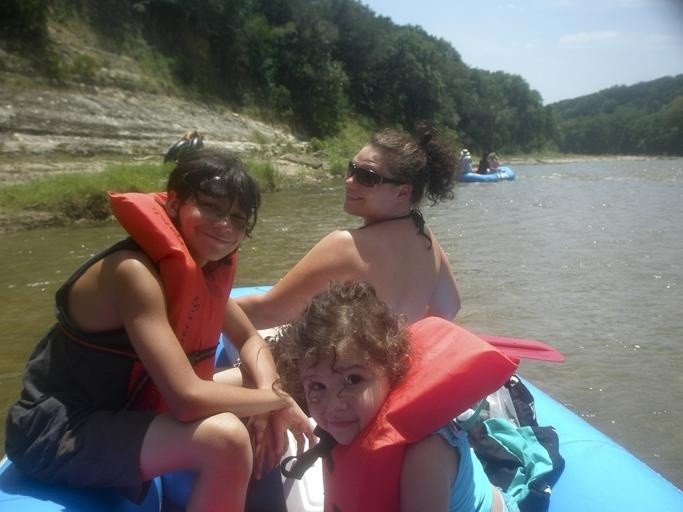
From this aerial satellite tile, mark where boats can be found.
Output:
[456,166,515,182]
[2,283,683,511]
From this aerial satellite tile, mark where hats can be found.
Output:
[461,149,470,156]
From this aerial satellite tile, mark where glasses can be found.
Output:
[199,176,250,210]
[348,160,409,187]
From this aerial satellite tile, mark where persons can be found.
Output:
[459,149,474,173]
[488,152,500,172]
[5,130,320,512]
[476,150,491,174]
[229,122,461,412]
[263,278,525,512]
